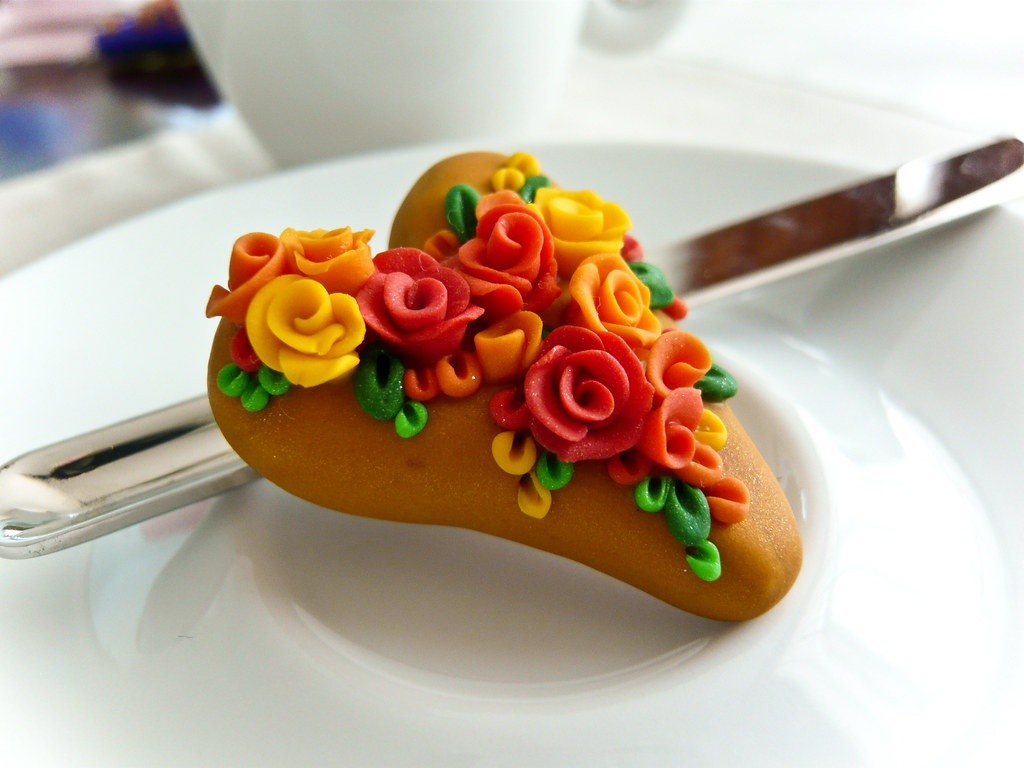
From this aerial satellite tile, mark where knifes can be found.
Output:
[0,138,1024,558]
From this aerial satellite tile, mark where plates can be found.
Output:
[0,149,1024,768]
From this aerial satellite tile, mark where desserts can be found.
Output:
[205,150,802,622]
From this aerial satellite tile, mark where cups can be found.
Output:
[169,1,680,170]
